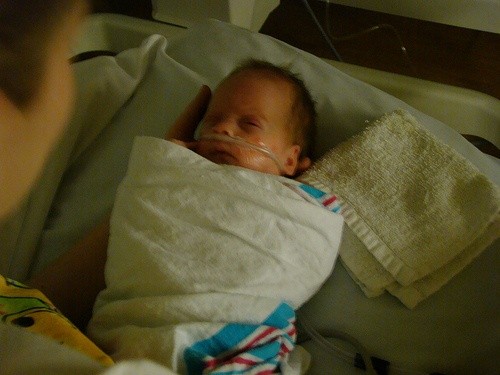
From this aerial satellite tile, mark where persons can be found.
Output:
[0,0,210,373]
[106,54,318,375]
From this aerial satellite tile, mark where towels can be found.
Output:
[296,109,500,309]
